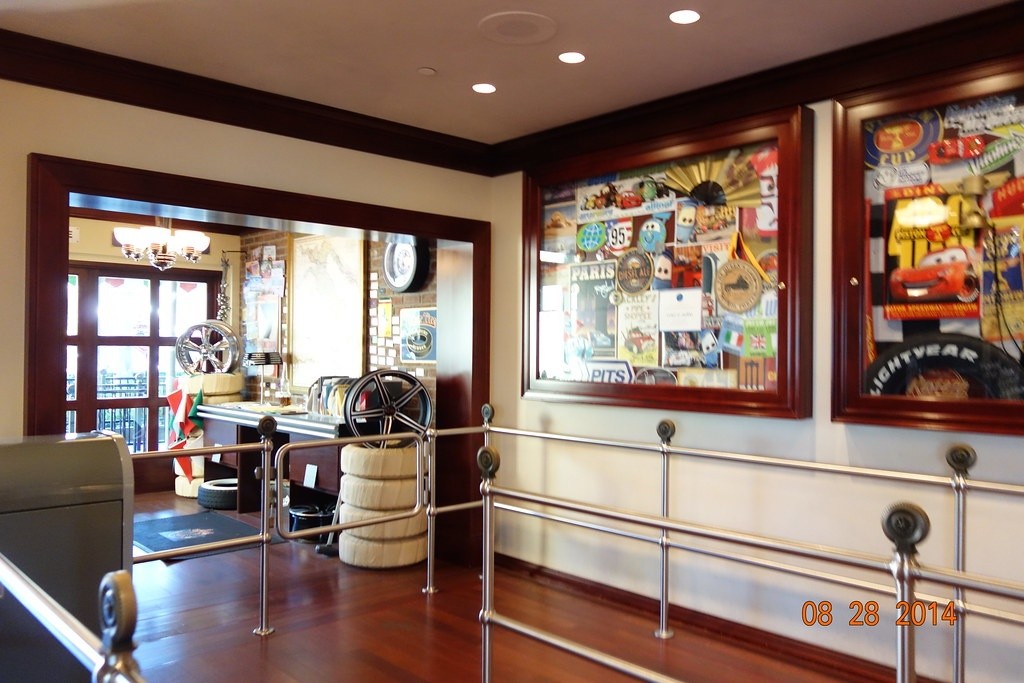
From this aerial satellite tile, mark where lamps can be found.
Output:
[114,225,210,271]
[243,351,282,407]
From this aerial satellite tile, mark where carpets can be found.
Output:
[133,509,289,562]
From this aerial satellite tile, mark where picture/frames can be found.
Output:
[286,225,367,394]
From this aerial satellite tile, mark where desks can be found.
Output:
[196,401,367,532]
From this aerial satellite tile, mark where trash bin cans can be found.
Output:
[0,429,136,683]
